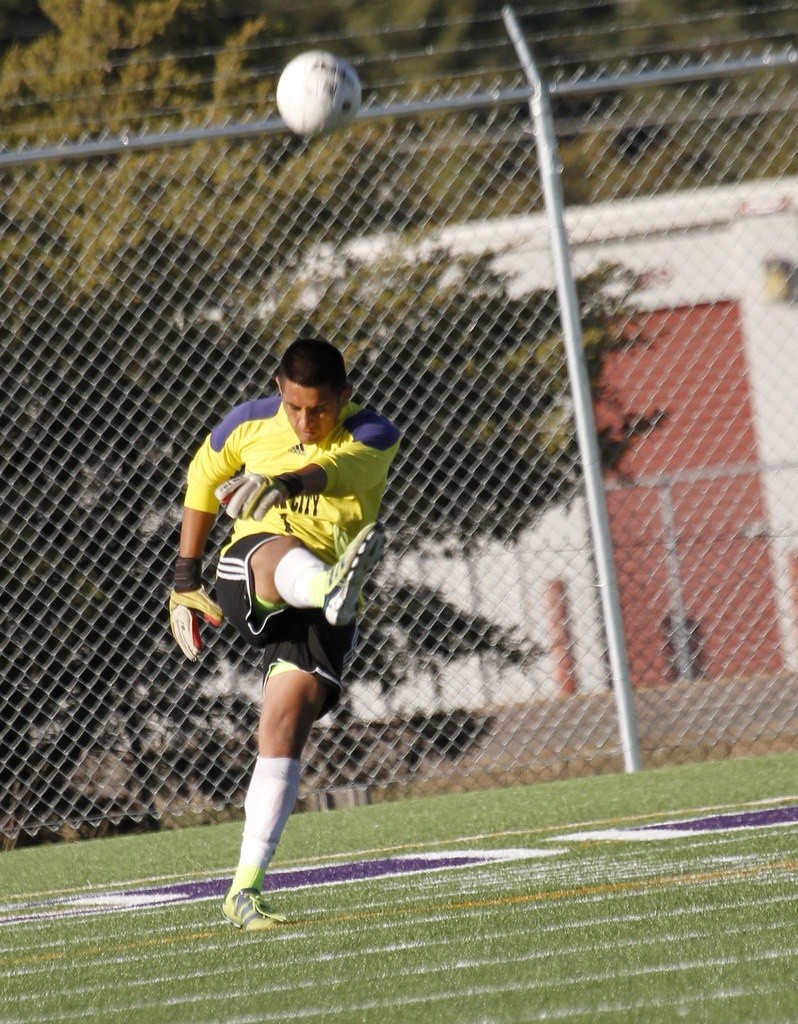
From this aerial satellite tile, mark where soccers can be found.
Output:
[276,49,362,138]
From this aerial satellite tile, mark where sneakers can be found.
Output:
[220,884,290,931]
[323,517,387,625]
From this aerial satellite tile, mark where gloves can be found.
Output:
[215,468,304,521]
[167,559,223,662]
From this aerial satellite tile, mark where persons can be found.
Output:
[169,339,402,931]
